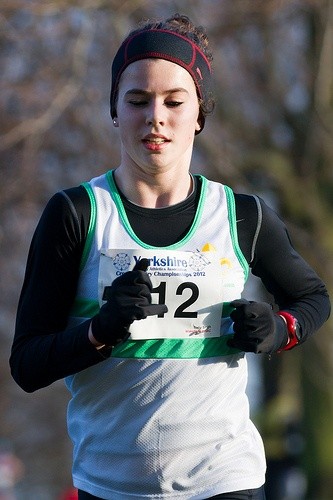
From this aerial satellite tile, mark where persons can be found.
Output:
[8,14,333,500]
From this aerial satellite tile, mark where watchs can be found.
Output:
[278,310,302,350]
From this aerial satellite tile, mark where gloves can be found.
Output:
[224,294,290,357]
[86,254,169,344]
